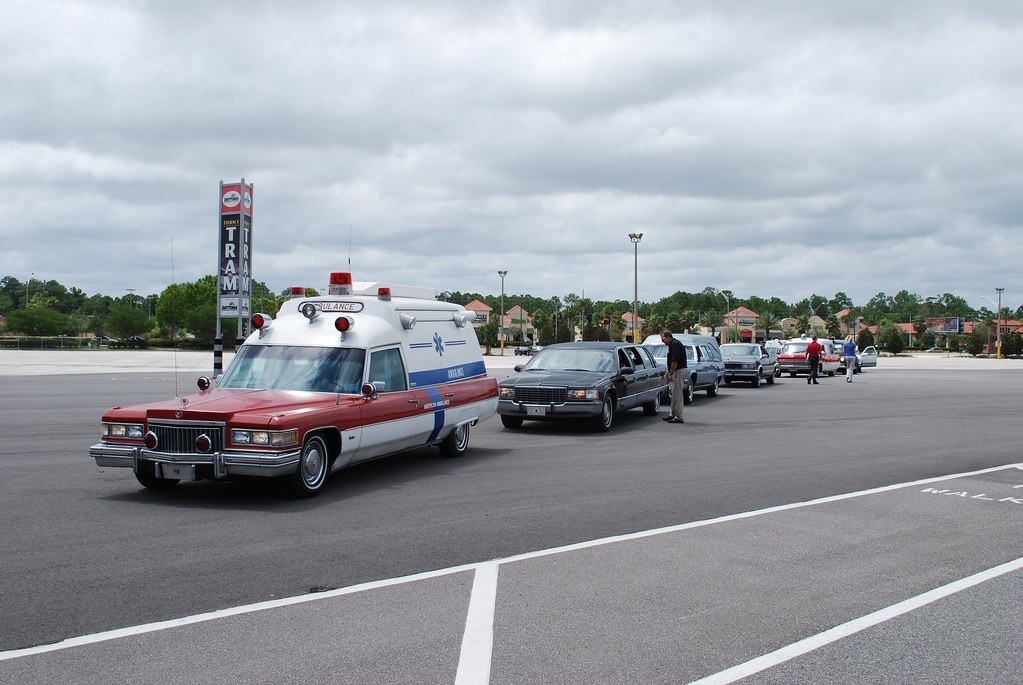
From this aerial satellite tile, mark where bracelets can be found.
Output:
[670,372,673,375]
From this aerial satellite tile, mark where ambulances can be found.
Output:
[88,273,499,501]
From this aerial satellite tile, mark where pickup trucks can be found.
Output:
[641,334,725,407]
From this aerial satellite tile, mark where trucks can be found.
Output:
[765,339,787,357]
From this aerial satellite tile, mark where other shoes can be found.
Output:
[663,416,674,421]
[807,377,811,384]
[846,377,852,382]
[813,381,819,384]
[668,417,684,423]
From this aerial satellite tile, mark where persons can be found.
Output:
[660,331,687,424]
[805,336,823,384]
[843,335,859,383]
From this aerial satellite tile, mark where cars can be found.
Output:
[925,347,943,353]
[89,335,118,346]
[719,343,777,388]
[833,340,877,375]
[777,334,840,377]
[122,336,146,348]
[526,346,543,357]
[497,342,667,433]
[514,346,527,356]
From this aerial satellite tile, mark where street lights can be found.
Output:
[629,233,643,344]
[995,287,1005,359]
[126,289,135,310]
[497,270,508,355]
